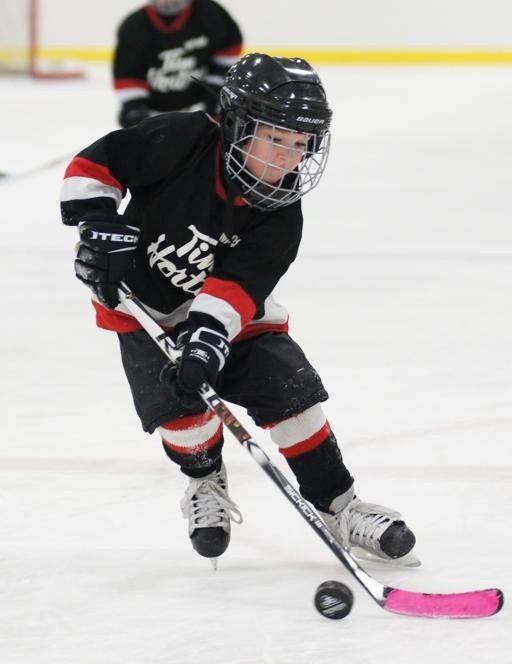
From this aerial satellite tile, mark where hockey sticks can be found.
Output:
[115,282,503,620]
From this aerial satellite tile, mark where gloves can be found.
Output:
[73,217,147,311]
[119,98,149,129]
[157,311,230,409]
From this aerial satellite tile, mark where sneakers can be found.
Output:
[297,475,416,561]
[179,462,243,559]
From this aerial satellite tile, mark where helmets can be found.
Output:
[218,52,334,212]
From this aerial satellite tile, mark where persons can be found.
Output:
[113,0,245,128]
[61,51,418,563]
